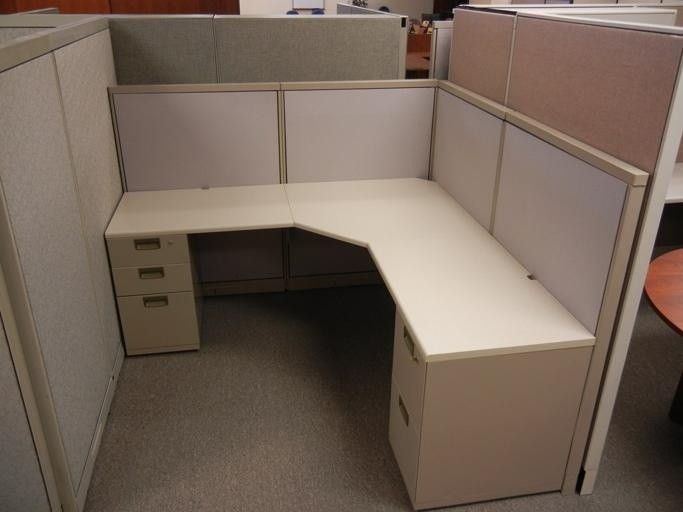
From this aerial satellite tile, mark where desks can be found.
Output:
[281,177,597,509]
[104,182,295,355]
